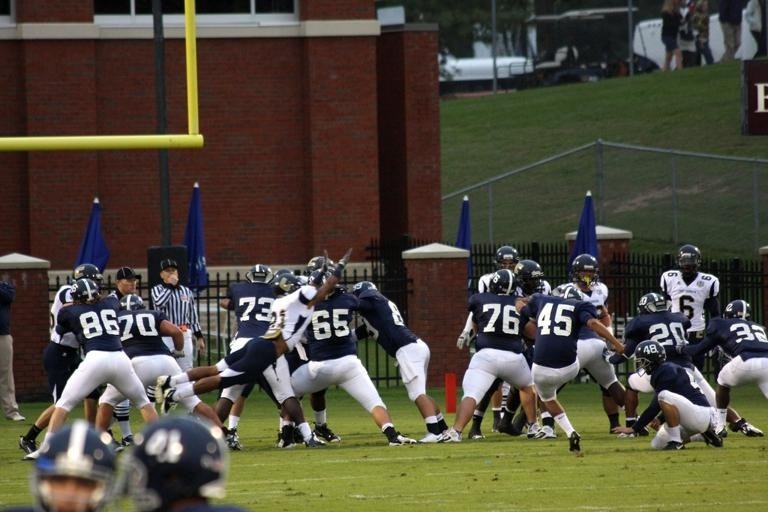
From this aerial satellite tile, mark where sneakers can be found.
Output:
[310,423,342,444]
[18,434,36,453]
[23,442,49,461]
[226,429,244,451]
[154,374,173,403]
[122,433,137,446]
[441,417,651,451]
[106,427,124,453]
[6,412,26,422]
[659,441,687,452]
[160,388,177,416]
[277,423,328,448]
[388,430,416,446]
[417,432,444,443]
[701,418,765,447]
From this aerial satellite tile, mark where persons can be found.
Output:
[437,246,768,452]
[1,273,27,421]
[18,248,453,461]
[10,418,119,511]
[120,413,252,510]
[661,0,767,73]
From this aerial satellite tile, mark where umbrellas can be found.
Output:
[453,196,473,300]
[566,189,598,274]
[181,181,208,294]
[75,198,109,275]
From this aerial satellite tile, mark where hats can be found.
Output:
[114,265,143,281]
[158,258,179,273]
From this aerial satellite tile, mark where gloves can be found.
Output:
[674,339,690,355]
[337,246,354,269]
[321,246,329,274]
[171,347,185,359]
[601,347,621,364]
[456,331,473,350]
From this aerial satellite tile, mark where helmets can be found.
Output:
[70,278,101,301]
[489,268,518,297]
[557,285,584,302]
[636,291,673,315]
[33,422,121,512]
[126,412,230,504]
[307,269,337,296]
[571,252,602,284]
[73,262,104,280]
[351,280,378,295]
[119,292,146,313]
[676,244,703,269]
[244,264,275,285]
[271,268,305,298]
[494,245,519,263]
[722,299,753,321]
[306,255,337,278]
[633,338,668,376]
[513,258,545,297]
